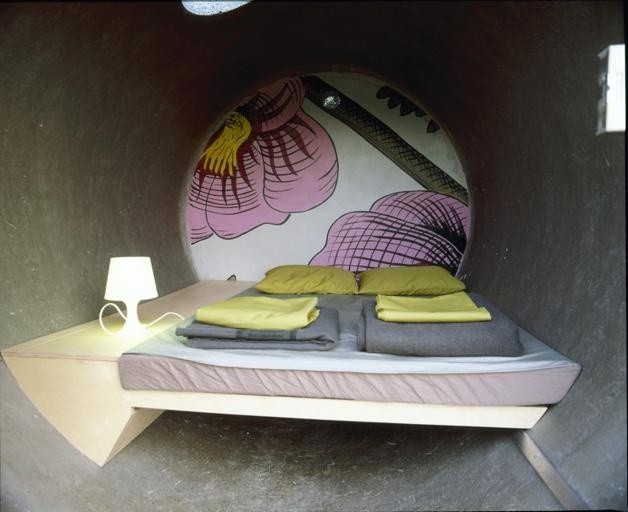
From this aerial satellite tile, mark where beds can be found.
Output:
[116,283,584,431]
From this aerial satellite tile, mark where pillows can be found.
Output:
[254,265,467,296]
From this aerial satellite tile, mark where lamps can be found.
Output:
[98,256,159,344]
